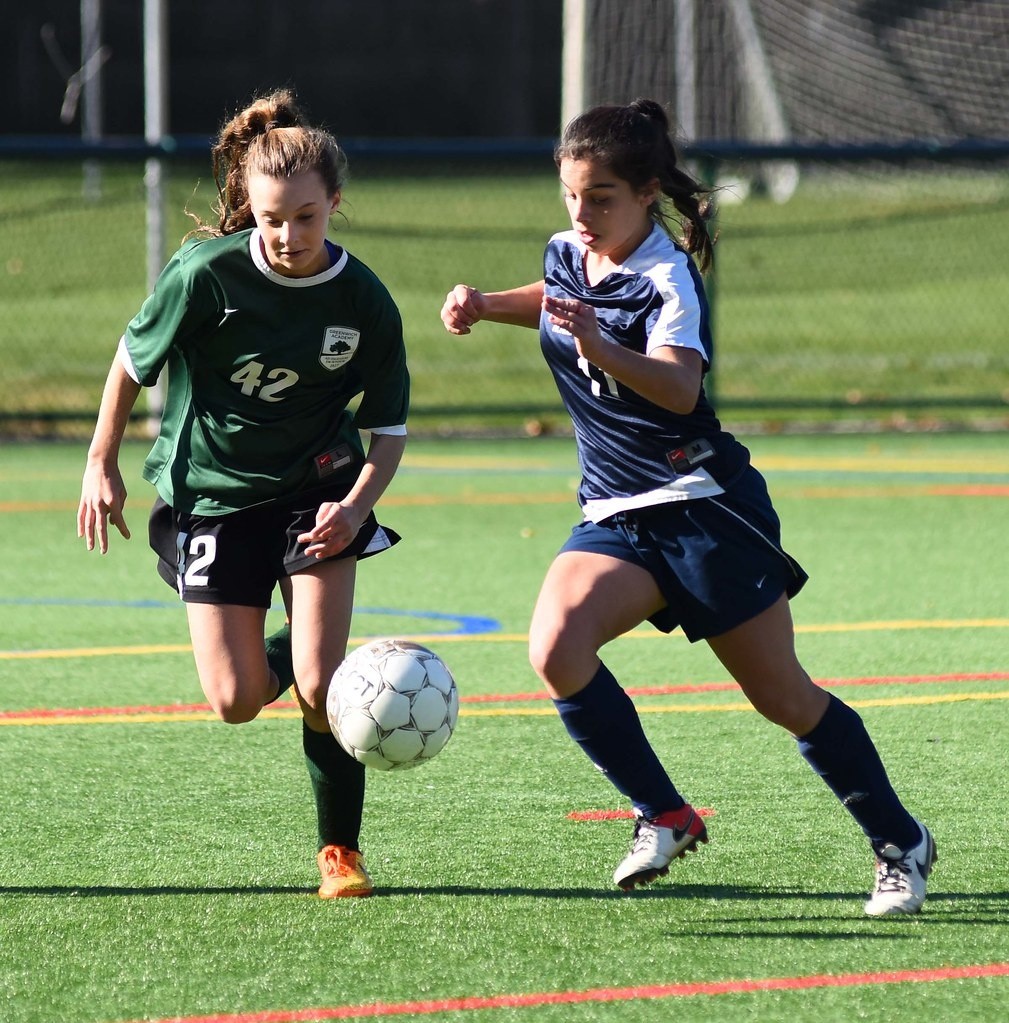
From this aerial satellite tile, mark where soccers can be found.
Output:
[324,636,460,774]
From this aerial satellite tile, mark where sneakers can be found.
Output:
[864,823,936,920]
[315,845,372,900]
[614,802,709,892]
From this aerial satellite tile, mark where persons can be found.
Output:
[75,88,409,898]
[442,98,938,917]
